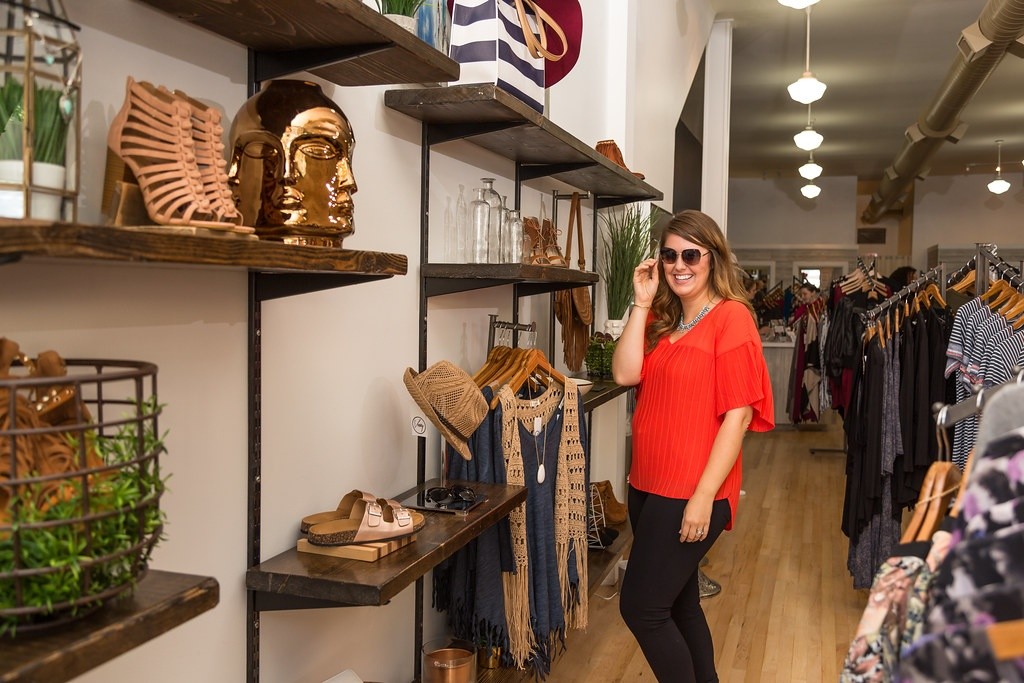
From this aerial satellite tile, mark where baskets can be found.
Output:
[0,356,161,640]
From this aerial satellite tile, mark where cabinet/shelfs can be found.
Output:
[0,0,664,683]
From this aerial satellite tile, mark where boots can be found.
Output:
[596,140,646,181]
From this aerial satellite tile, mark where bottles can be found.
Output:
[467,178,525,264]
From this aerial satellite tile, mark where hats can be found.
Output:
[403,360,490,461]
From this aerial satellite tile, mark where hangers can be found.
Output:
[898,404,960,543]
[471,320,504,382]
[490,324,552,410]
[475,321,514,388]
[480,323,523,390]
[475,320,505,383]
[836,241,1024,349]
[489,323,541,396]
[948,386,988,519]
[511,327,565,396]
[915,404,963,541]
[753,285,828,324]
[986,618,1024,661]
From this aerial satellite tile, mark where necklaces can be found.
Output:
[524,364,551,483]
[677,292,717,332]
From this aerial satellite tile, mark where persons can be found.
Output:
[743,278,757,300]
[221,78,357,237]
[610,207,776,683]
[887,267,918,292]
[799,283,818,303]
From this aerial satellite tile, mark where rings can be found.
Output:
[696,530,704,535]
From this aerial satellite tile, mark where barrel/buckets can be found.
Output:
[421,637,477,683]
[478,646,502,668]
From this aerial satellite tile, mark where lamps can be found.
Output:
[793,104,824,150]
[862,211,871,222]
[903,122,926,146]
[914,164,932,181]
[987,140,1010,194]
[786,4,827,106]
[800,179,822,199]
[956,20,994,63]
[797,150,823,181]
[945,121,972,145]
[884,165,898,182]
[871,192,881,205]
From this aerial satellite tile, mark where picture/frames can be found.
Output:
[857,228,886,244]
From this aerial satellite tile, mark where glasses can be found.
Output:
[426,484,477,504]
[658,246,711,267]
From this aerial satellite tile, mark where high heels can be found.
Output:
[522,215,551,266]
[540,218,568,268]
[172,87,253,234]
[99,76,237,231]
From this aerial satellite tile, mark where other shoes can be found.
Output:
[698,569,721,598]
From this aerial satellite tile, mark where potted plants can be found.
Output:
[0,77,76,223]
[0,358,173,640]
[375,0,427,37]
[586,200,668,340]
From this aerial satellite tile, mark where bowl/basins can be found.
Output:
[567,377,593,396]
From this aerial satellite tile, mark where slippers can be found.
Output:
[308,500,425,547]
[300,488,418,534]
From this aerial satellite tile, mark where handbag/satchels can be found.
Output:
[448,0,569,115]
[553,193,594,373]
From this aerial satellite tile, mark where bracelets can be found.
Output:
[630,301,652,309]
